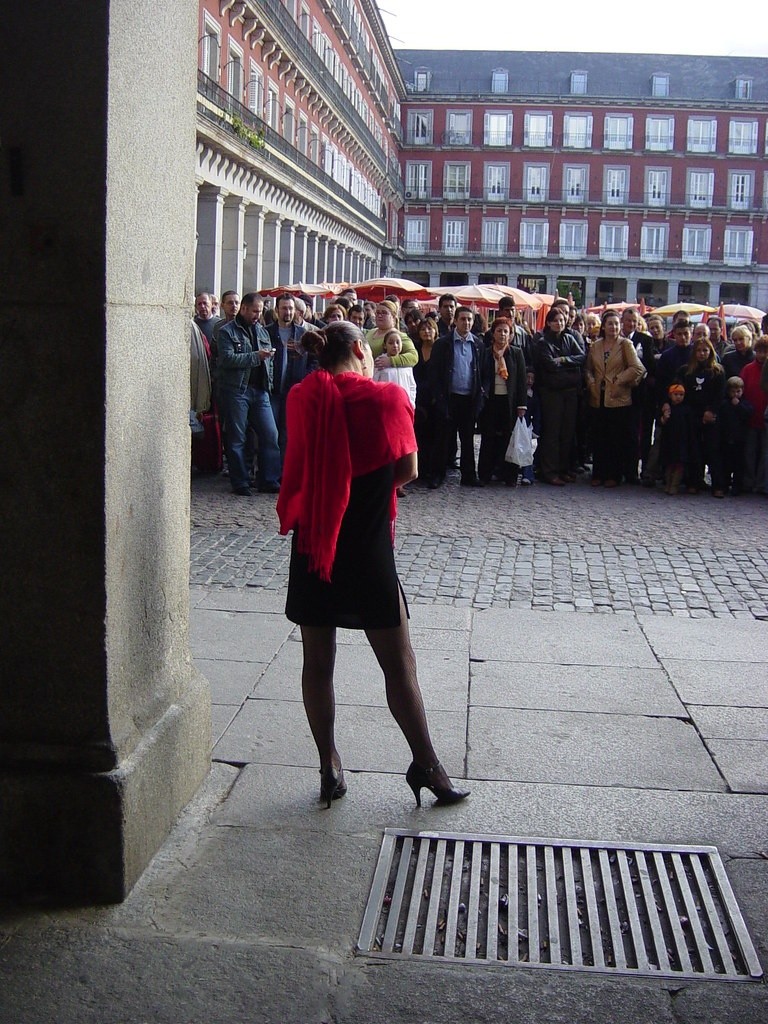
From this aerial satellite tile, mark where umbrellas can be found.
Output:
[258,276,768,342]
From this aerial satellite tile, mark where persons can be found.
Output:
[190,288,768,496]
[276,321,471,808]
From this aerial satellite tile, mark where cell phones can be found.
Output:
[270,348,276,352]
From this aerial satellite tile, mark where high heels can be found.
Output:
[406,760,471,807]
[319,765,348,809]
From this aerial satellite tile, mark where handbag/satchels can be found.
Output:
[621,338,644,387]
[505,414,538,467]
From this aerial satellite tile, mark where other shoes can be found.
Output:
[591,478,602,486]
[583,456,595,465]
[231,487,252,496]
[220,465,229,476]
[567,469,577,477]
[460,477,485,487]
[257,482,280,494]
[428,472,440,488]
[602,477,619,489]
[397,487,407,496]
[543,472,565,486]
[624,461,768,497]
[505,481,516,487]
[558,473,571,482]
[581,463,591,471]
[521,478,531,485]
[571,461,585,473]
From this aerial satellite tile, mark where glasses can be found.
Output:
[374,310,394,317]
